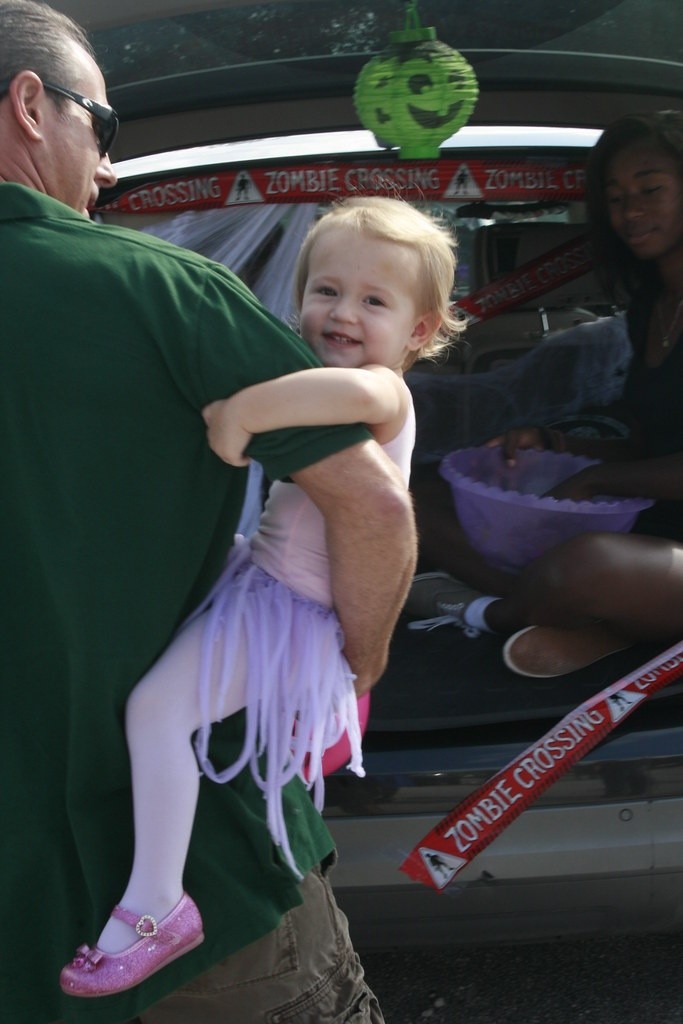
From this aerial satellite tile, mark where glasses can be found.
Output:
[0,80,121,159]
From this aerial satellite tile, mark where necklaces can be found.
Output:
[653,298,681,350]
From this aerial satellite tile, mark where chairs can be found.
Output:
[461,221,602,373]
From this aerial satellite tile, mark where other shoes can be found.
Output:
[59,891,205,998]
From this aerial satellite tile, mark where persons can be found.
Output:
[58,197,453,997]
[0,1,419,1024]
[399,119,682,677]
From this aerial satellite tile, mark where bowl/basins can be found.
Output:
[438,441,654,572]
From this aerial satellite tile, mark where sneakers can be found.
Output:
[403,572,487,639]
[503,625,645,678]
